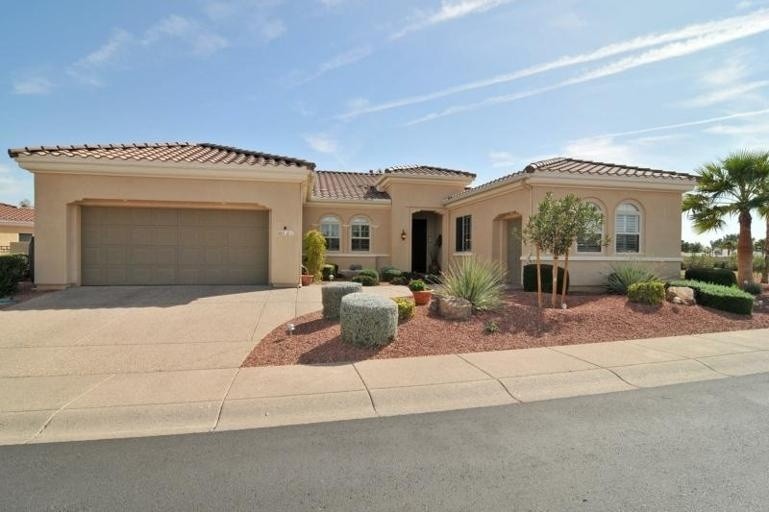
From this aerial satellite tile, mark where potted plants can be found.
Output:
[407,279,434,306]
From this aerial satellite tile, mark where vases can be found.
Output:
[302,273,315,285]
